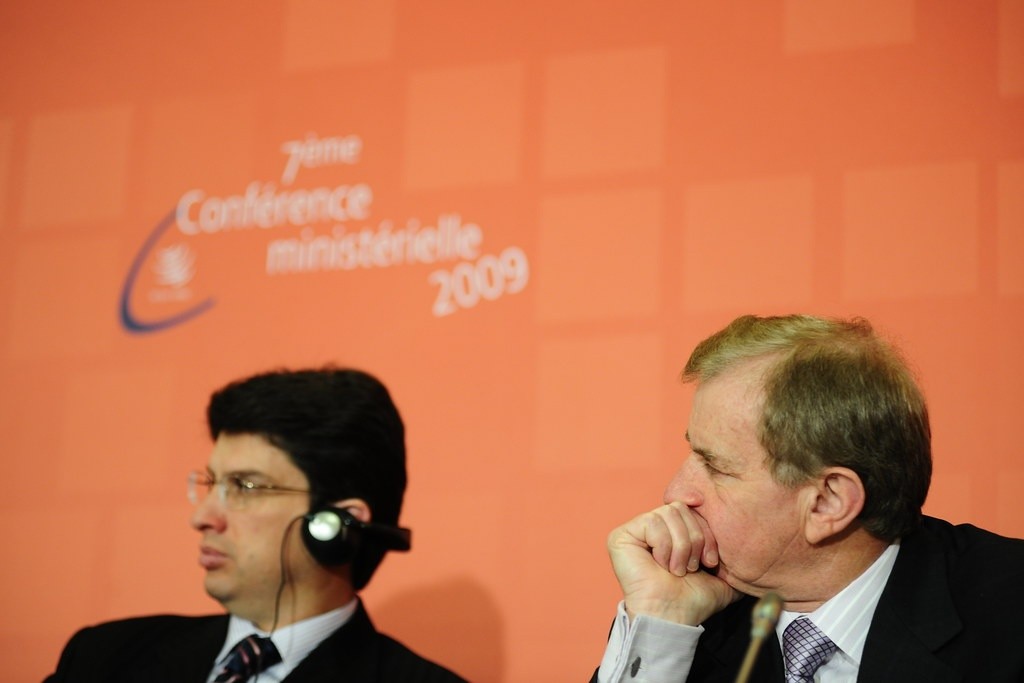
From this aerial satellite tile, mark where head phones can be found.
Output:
[299,504,411,558]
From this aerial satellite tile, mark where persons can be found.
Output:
[42,367,469,683]
[589,314,1024,683]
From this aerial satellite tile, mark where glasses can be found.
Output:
[187,469,314,506]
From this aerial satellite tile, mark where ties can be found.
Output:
[214,634,282,683]
[782,619,837,683]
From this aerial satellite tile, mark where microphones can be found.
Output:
[735,592,785,683]
